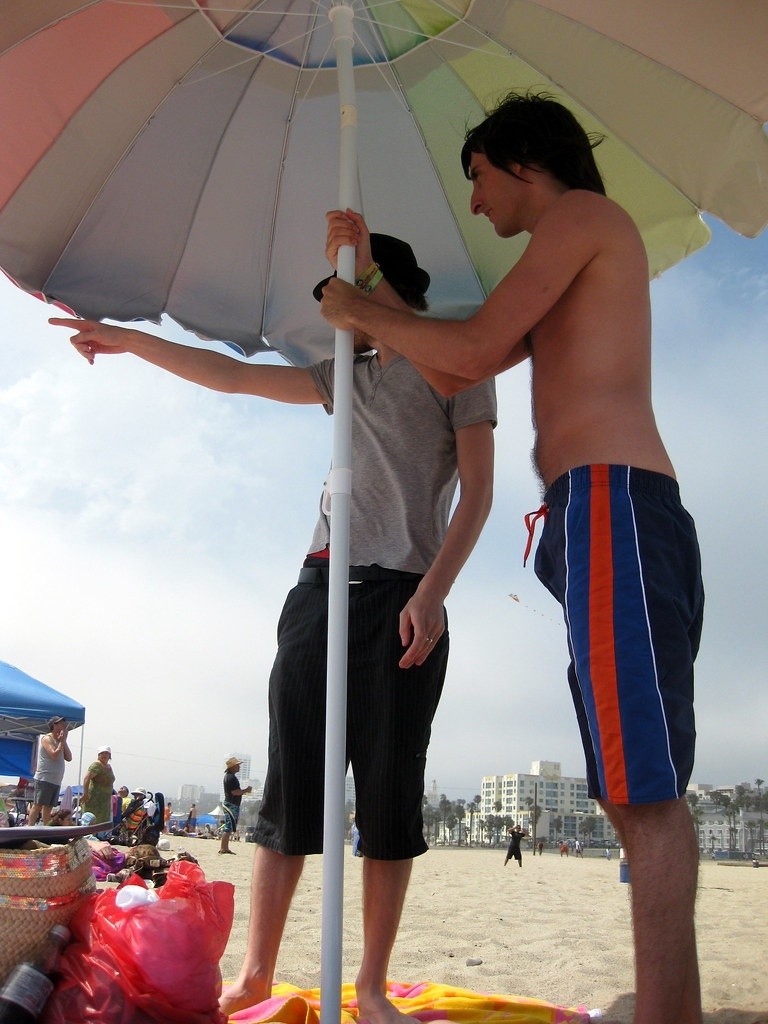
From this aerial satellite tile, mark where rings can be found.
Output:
[88,345,92,352]
[427,638,433,642]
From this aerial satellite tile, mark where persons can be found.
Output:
[117,785,131,815]
[574,836,583,859]
[537,841,544,856]
[559,841,568,857]
[214,819,226,837]
[28,716,72,826]
[43,809,71,844]
[205,823,210,833]
[606,847,611,860]
[504,825,526,867]
[187,803,196,833]
[319,94,706,1024]
[78,812,101,842]
[79,744,115,825]
[711,851,716,861]
[48,231,495,1024]
[218,757,252,854]
[164,802,173,832]
[127,787,156,839]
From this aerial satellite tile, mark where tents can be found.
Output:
[0,660,86,825]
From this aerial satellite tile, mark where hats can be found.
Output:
[224,756,243,772]
[48,715,65,732]
[97,746,111,756]
[132,788,146,798]
[312,234,431,302]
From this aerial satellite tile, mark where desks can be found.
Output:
[9,797,40,825]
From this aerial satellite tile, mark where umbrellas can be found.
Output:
[0,0,768,1024]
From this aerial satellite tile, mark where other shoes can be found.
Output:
[219,848,237,855]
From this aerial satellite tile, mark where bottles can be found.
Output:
[0,924,70,1023]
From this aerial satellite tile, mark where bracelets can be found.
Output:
[355,263,385,301]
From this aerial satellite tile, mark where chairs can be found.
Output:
[99,791,157,847]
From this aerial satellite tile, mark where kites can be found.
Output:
[508,593,567,630]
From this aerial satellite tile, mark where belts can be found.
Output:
[298,568,422,586]
